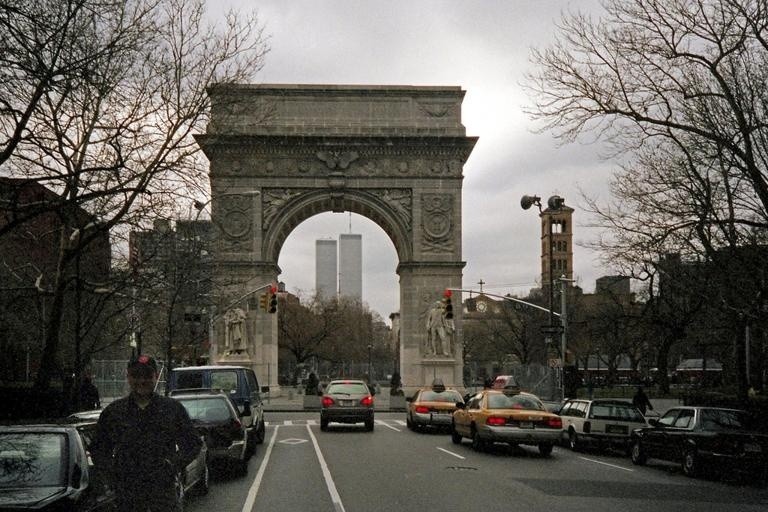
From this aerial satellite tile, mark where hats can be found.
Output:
[127,355,158,373]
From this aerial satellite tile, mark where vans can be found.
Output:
[319,374,330,379]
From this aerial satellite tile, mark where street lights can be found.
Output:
[520,195,565,324]
[189,192,262,263]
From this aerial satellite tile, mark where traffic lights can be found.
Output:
[444,289,456,319]
[259,285,277,313]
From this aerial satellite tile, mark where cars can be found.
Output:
[318,379,375,431]
[0,387,250,510]
[630,406,767,480]
[451,389,565,456]
[405,387,466,432]
[561,353,726,388]
[553,398,650,455]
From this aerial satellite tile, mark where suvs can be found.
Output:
[165,365,270,444]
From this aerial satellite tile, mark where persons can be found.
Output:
[88,353,201,511]
[381,188,412,232]
[260,188,302,227]
[426,300,451,358]
[632,385,654,418]
[79,375,100,411]
[306,371,319,395]
[221,298,250,357]
